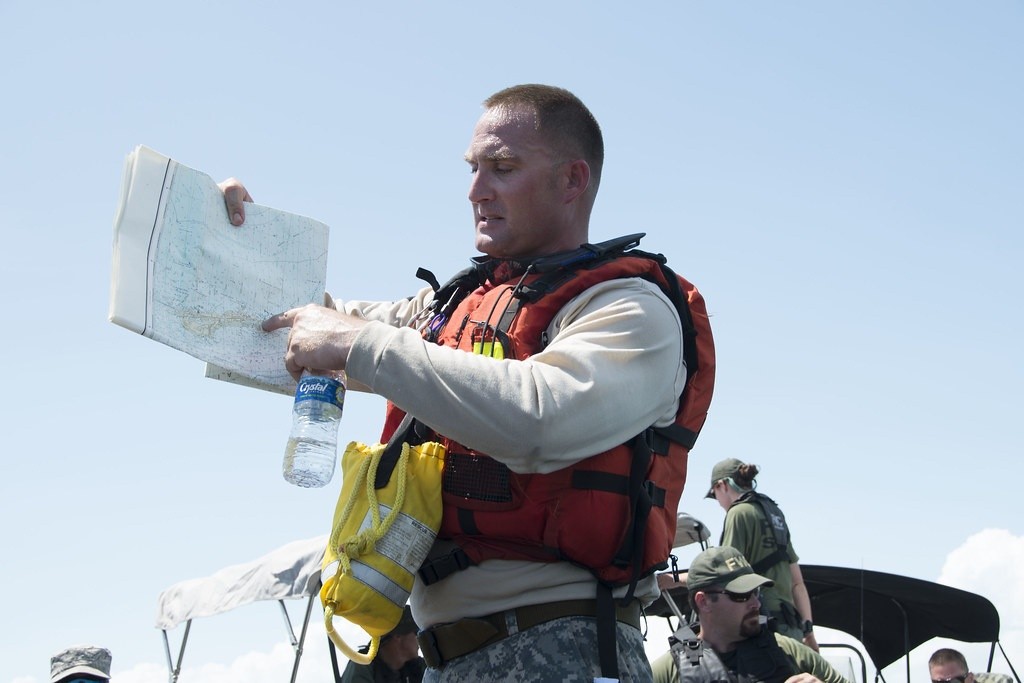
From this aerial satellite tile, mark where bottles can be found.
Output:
[284,368,347,487]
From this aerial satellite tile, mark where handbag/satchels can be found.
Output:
[320,441,446,665]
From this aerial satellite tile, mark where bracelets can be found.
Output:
[801,620,814,633]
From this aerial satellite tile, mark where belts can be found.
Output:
[418,598,643,670]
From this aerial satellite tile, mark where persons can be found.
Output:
[929,649,1013,683]
[51,645,112,683]
[703,458,820,653]
[215,83,717,682]
[651,546,852,683]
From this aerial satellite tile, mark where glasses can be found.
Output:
[705,485,716,499]
[701,586,760,602]
[54,674,109,683]
[932,675,968,683]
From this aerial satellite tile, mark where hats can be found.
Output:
[686,546,775,594]
[703,457,754,499]
[49,646,112,683]
